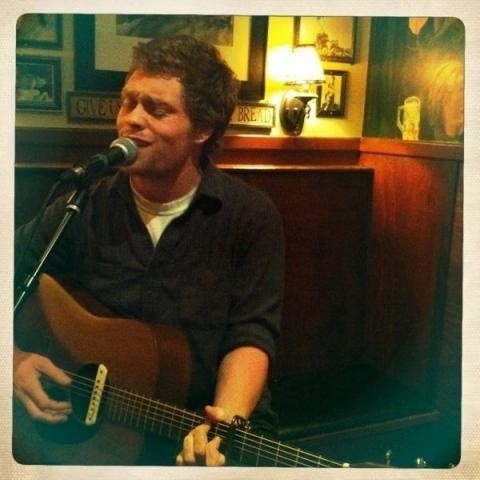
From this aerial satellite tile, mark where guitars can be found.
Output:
[13,274,455,468]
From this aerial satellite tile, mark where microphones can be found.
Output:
[59,137,137,183]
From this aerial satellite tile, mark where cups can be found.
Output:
[396,96,421,141]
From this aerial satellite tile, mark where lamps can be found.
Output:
[281,42,327,136]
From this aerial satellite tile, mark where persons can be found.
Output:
[13,34,286,466]
[322,92,338,112]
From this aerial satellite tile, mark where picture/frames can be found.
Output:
[16,13,63,50]
[315,68,348,120]
[295,15,357,64]
[74,15,269,104]
[16,55,63,115]
